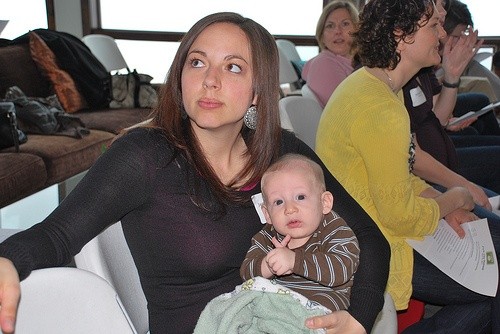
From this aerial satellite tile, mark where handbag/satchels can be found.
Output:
[0,103,27,152]
[108,69,159,107]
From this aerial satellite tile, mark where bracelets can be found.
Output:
[442,79,462,86]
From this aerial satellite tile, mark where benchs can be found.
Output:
[0,30,162,212]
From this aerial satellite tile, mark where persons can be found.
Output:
[434,0,500,136]
[189,153,361,334]
[401,0,499,215]
[0,11,391,334]
[314,0,500,334]
[301,0,500,194]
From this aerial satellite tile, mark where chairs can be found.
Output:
[0,265,138,334]
[276,48,301,94]
[300,83,325,108]
[79,34,130,74]
[276,89,323,154]
[275,37,304,61]
